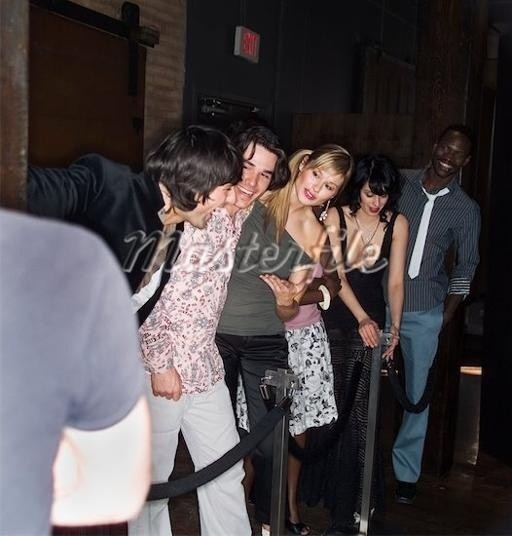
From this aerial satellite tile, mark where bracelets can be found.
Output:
[317,284,331,310]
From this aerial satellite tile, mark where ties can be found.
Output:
[407,187,452,279]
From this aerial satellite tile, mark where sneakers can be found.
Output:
[396,481,417,505]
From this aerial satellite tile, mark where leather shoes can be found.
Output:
[307,485,321,507]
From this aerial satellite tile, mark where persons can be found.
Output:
[214,143,355,536]
[234,259,340,535]
[382,124,480,506]
[127,120,293,536]
[295,152,410,535]
[27,124,244,328]
[0,208,154,536]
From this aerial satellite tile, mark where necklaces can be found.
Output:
[354,216,380,250]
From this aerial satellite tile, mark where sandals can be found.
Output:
[286,515,311,535]
